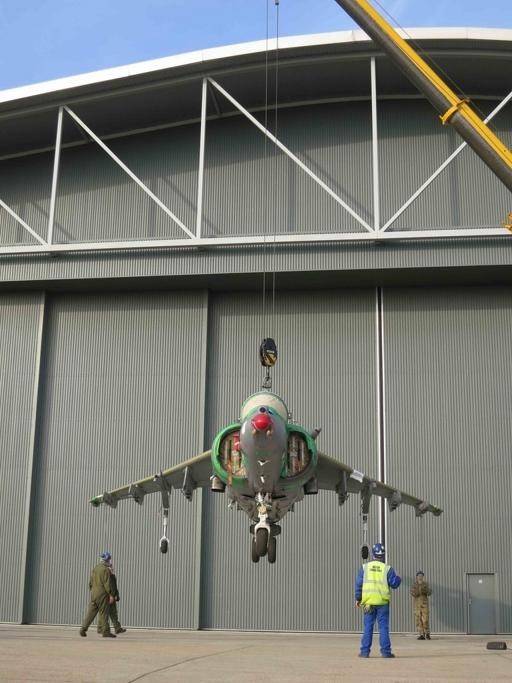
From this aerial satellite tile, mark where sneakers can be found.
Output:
[77,626,127,641]
[417,634,431,641]
[358,653,395,659]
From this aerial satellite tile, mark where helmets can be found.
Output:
[371,541,386,558]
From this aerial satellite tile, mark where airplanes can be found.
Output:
[89,391,444,563]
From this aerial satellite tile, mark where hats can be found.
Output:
[101,552,112,561]
[416,571,424,577]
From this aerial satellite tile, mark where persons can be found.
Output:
[79,551,120,638]
[409,570,432,640]
[97,561,127,635]
[353,541,401,658]
